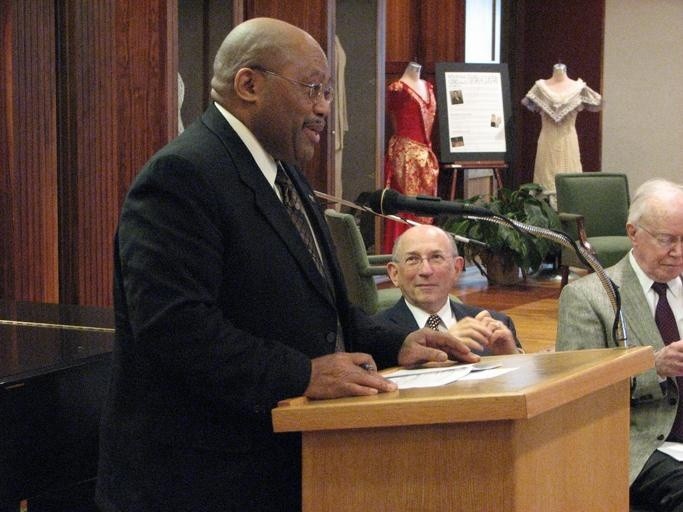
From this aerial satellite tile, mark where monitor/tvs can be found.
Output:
[434,61,518,162]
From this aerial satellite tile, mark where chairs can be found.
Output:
[322,206,464,353]
[554,170,633,292]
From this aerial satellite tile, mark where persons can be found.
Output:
[521,63,602,213]
[556,179,683,512]
[370,223,523,355]
[95,15,481,511]
[379,62,439,255]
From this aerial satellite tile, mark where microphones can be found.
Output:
[371,190,494,217]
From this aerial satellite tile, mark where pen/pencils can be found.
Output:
[359,363,375,372]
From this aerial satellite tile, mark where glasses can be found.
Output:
[396,254,454,267]
[638,226,682,248]
[258,69,334,105]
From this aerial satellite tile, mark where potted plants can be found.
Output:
[428,180,564,288]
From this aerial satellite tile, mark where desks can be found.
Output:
[0,299,117,511]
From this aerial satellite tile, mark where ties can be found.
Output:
[426,315,441,331]
[649,281,682,441]
[273,166,347,353]
